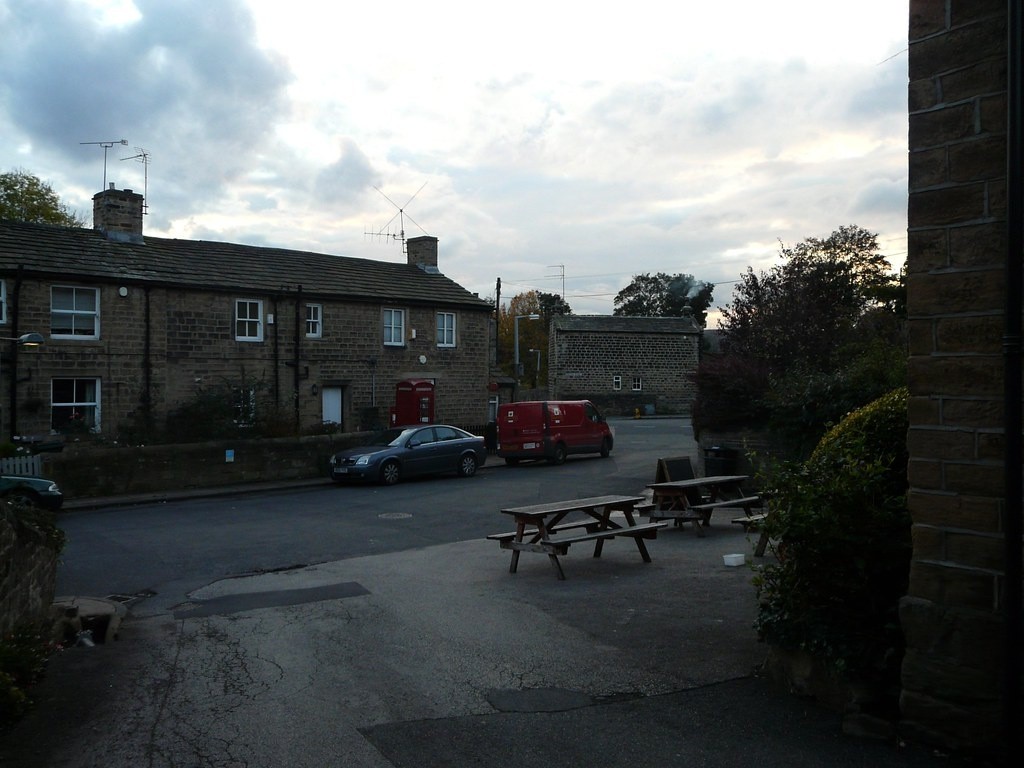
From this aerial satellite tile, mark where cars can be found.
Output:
[0,475,63,514]
[329,423,487,486]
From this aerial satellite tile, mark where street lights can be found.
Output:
[514,314,540,402]
[529,349,540,390]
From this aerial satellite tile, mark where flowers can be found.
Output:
[68,413,86,424]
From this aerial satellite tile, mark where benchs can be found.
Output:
[633,501,674,517]
[688,496,763,520]
[540,523,668,554]
[486,519,599,549]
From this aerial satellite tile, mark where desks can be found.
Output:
[500,495,652,581]
[646,476,751,539]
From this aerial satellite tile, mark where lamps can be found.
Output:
[312,384,319,396]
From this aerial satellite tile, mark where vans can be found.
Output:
[495,399,613,467]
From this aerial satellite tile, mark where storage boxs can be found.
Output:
[723,554,745,567]
